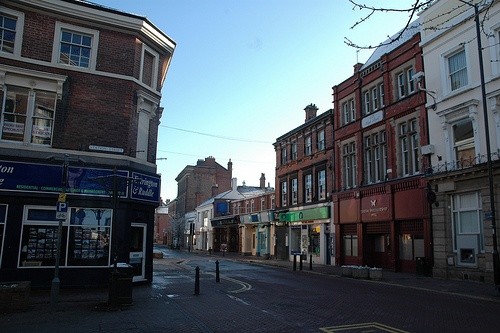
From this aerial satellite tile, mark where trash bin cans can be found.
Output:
[109,263,134,304]
[414,257,426,273]
